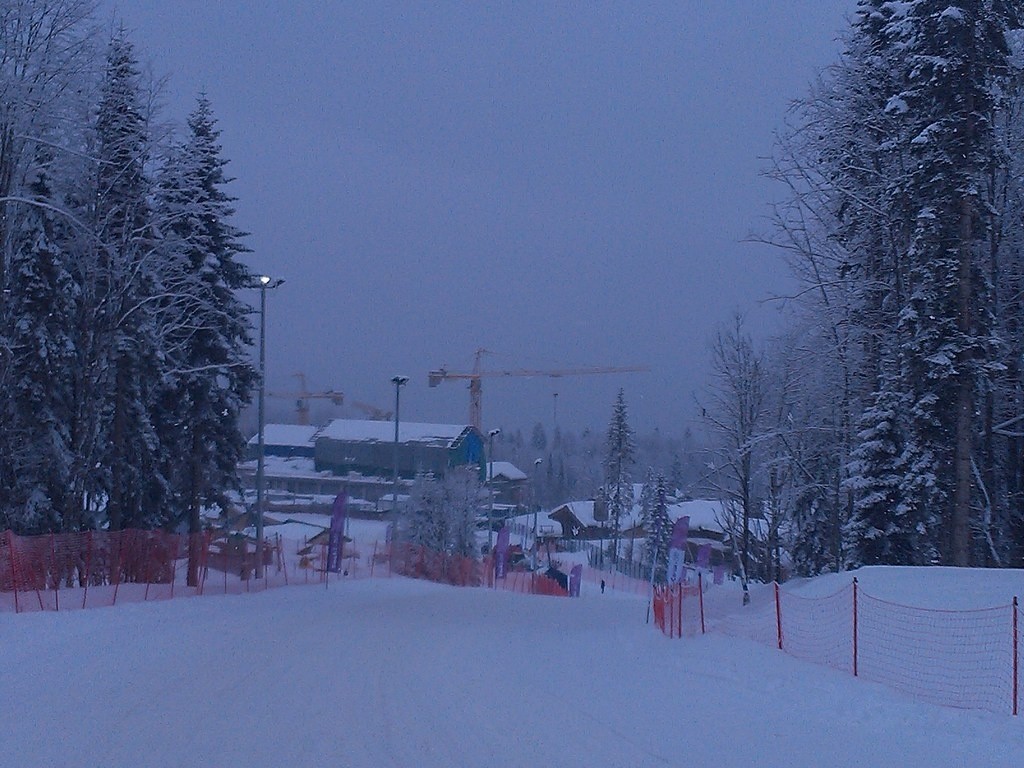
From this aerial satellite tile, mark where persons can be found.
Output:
[601,580,606,594]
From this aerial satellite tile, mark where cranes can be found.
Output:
[425,346,654,437]
[246,372,344,425]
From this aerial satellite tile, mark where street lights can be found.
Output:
[246,273,288,581]
[389,373,409,580]
[486,428,501,588]
[532,456,543,595]
